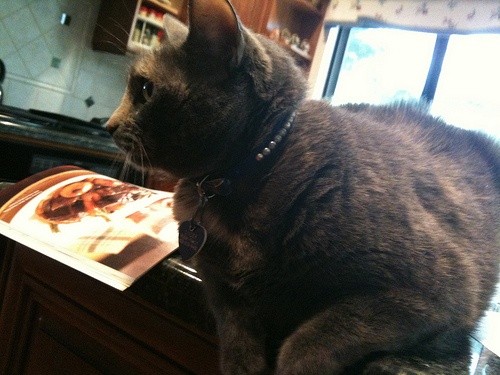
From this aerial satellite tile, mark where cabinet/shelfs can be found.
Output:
[90,1,332,84]
[0,234,222,375]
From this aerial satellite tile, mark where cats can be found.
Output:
[101,0,500,375]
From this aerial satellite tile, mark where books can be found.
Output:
[0,164,183,294]
